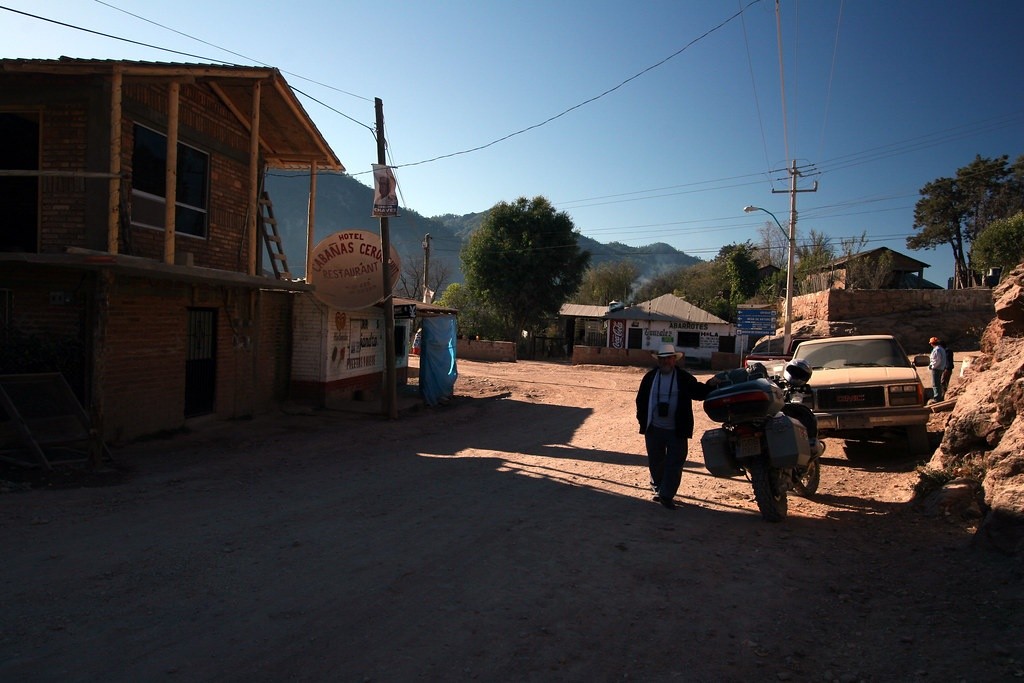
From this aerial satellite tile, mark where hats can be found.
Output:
[651,345,683,361]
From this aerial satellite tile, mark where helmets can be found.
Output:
[929,337,942,344]
[783,359,812,387]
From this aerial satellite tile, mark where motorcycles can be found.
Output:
[700,361,827,524]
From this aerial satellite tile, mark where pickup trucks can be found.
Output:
[742,334,835,370]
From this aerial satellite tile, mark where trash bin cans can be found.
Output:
[948,277,954,289]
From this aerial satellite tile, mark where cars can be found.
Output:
[793,334,932,455]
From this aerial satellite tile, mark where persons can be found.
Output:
[374,176,396,205]
[928,337,954,402]
[635,345,721,510]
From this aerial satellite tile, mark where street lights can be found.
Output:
[744,205,797,355]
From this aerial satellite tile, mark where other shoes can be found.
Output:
[663,502,676,510]
[653,494,659,501]
